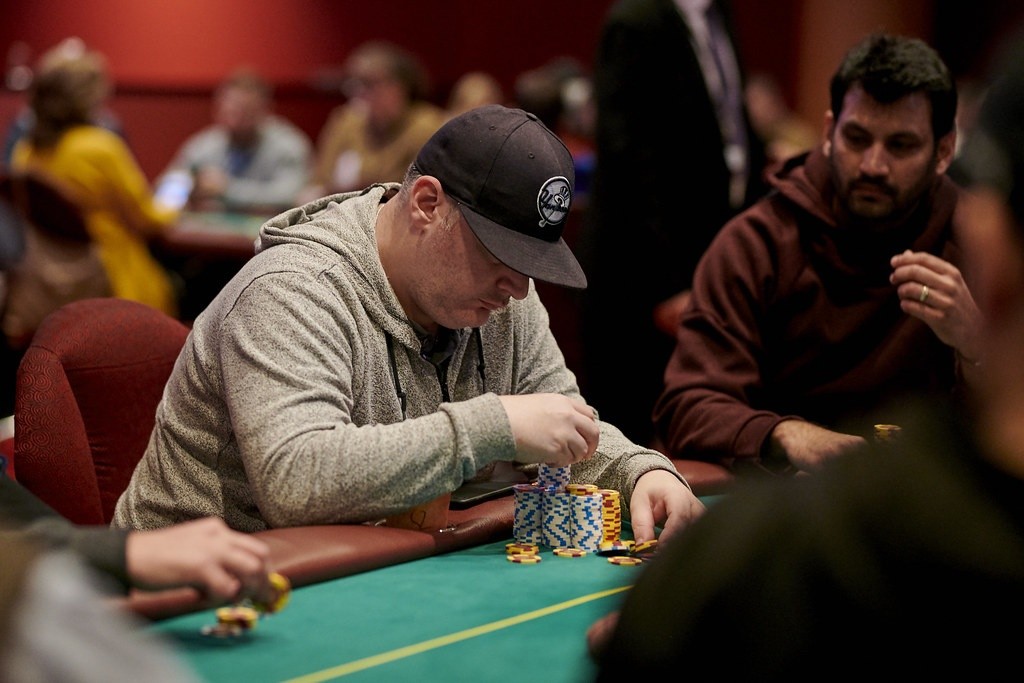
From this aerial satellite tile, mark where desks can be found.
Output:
[98,459,749,683]
[146,207,278,279]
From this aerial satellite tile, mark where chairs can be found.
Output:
[14,294,199,532]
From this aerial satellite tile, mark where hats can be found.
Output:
[414,107,587,290]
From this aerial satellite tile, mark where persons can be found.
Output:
[1,468,274,602]
[107,104,706,551]
[588,66,1024,682]
[297,45,442,205]
[12,37,181,315]
[653,34,992,468]
[156,80,311,211]
[586,0,766,444]
[747,81,817,159]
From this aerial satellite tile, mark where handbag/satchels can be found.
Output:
[4,247,113,341]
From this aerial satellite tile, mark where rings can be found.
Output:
[920,286,929,302]
